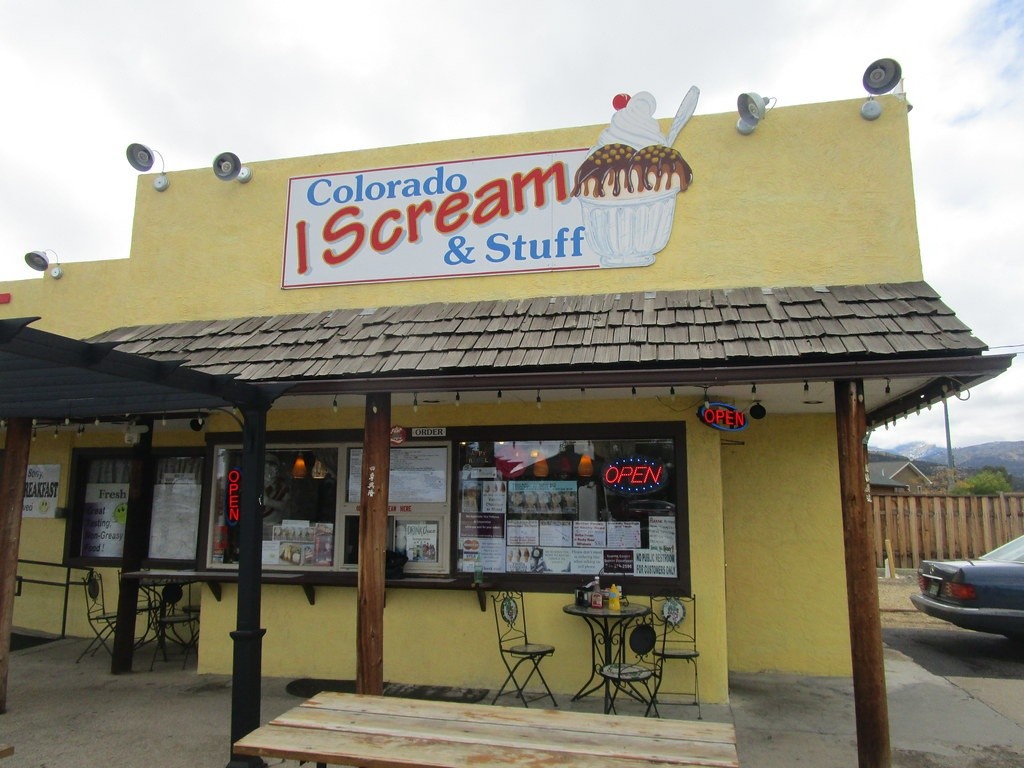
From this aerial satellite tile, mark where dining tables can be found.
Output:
[133,578,200,652]
[562,600,651,714]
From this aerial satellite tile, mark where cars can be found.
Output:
[910,535,1024,640]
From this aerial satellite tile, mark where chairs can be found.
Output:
[180,577,200,655]
[649,589,704,719]
[117,567,163,656]
[76,571,117,663]
[491,587,558,708]
[150,573,198,671]
[599,612,667,719]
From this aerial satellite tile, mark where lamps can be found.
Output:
[861,58,902,121]
[212,152,253,184]
[126,143,171,192]
[24,249,63,280]
[892,77,913,113]
[736,92,777,135]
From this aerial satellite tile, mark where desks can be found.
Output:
[232,690,739,768]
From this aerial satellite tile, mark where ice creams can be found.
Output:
[508,547,530,562]
[568,89,695,270]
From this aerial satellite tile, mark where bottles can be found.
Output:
[473,554,484,584]
[591,576,603,608]
[608,584,621,610]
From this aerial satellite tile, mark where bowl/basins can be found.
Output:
[573,175,694,271]
